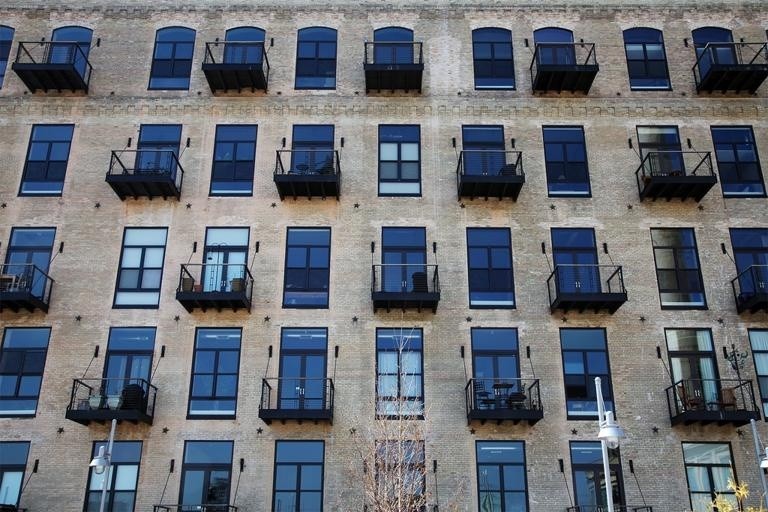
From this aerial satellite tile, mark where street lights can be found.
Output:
[588,375,628,512]
[92,419,117,512]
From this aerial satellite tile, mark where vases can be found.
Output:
[194,285,203,292]
[76,383,145,411]
[231,278,243,292]
[411,271,428,294]
[501,163,516,176]
[182,278,194,292]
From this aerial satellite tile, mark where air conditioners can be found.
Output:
[720,387,737,413]
[0,274,20,293]
